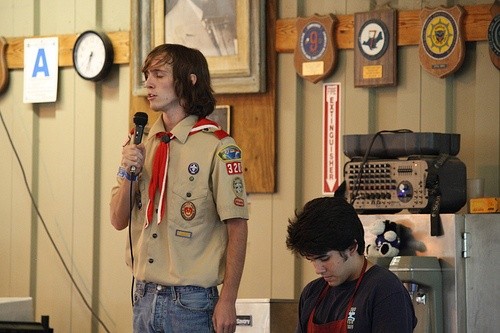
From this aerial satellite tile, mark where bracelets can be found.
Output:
[118,166,139,181]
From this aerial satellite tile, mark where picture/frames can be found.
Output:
[131,0,266,98]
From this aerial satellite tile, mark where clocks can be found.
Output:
[72,29,114,82]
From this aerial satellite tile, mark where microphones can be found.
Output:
[130,111,149,179]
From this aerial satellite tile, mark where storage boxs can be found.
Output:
[470,197,500,214]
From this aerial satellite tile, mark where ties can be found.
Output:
[145,115,228,230]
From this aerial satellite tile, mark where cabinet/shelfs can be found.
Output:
[233,298,300,333]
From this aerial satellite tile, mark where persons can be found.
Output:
[109,44,249,333]
[165,0,235,56]
[286,196,417,333]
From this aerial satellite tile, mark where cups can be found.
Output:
[466,178,484,214]
